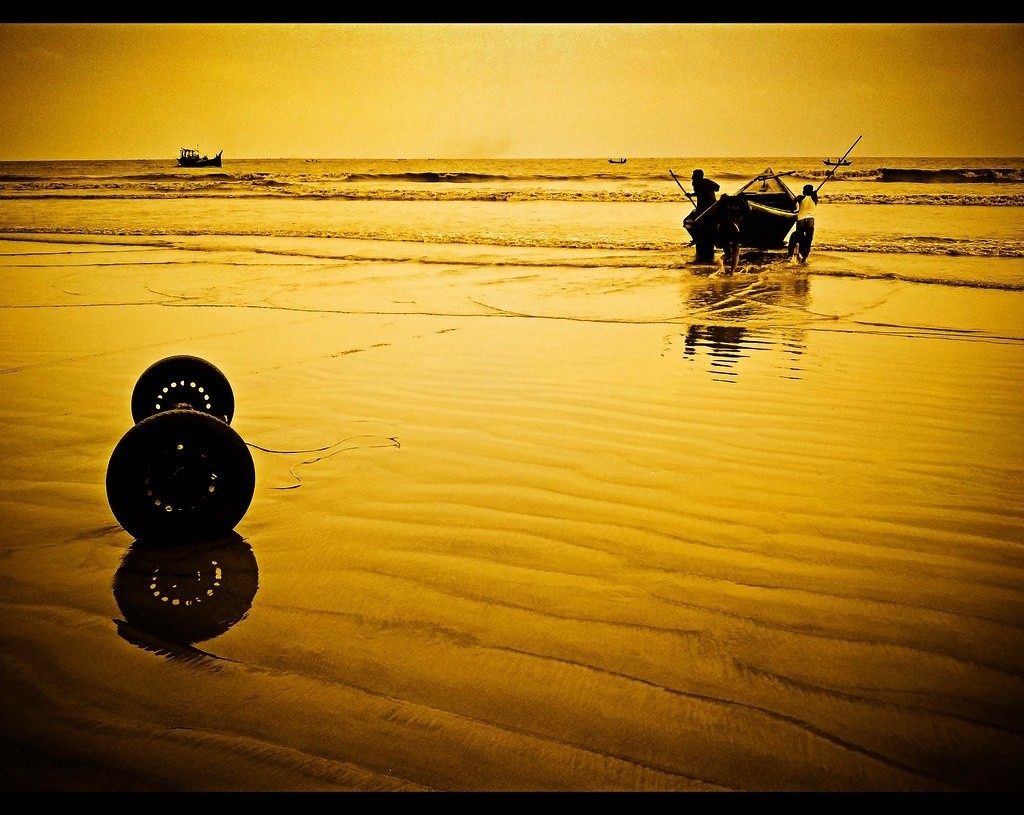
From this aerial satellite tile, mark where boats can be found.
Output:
[684,165,801,250]
[177,147,223,167]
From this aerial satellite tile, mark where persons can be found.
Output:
[792,184,819,264]
[715,193,751,278]
[683,170,720,248]
